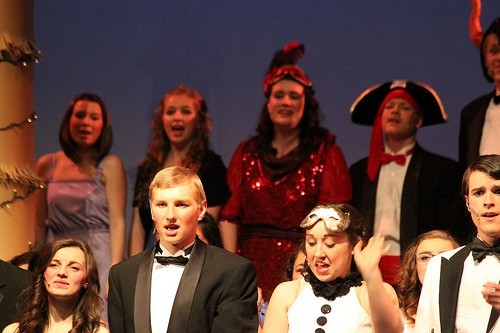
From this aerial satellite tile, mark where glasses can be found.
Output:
[416,253,436,262]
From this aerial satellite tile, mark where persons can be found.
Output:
[458,19,500,246]
[29,92,126,321]
[126,85,232,256]
[260,201,405,333]
[393,230,460,333]
[259,248,308,333]
[107,167,258,333]
[218,62,352,301]
[0,238,108,333]
[349,79,461,292]
[413,155,500,333]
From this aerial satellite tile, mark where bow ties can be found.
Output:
[470,237,500,263]
[493,96,500,104]
[154,240,194,266]
[381,146,415,166]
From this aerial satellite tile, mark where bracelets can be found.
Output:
[35,241,44,249]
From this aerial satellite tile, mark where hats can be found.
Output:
[480,19,500,83]
[264,41,313,97]
[350,80,448,127]
